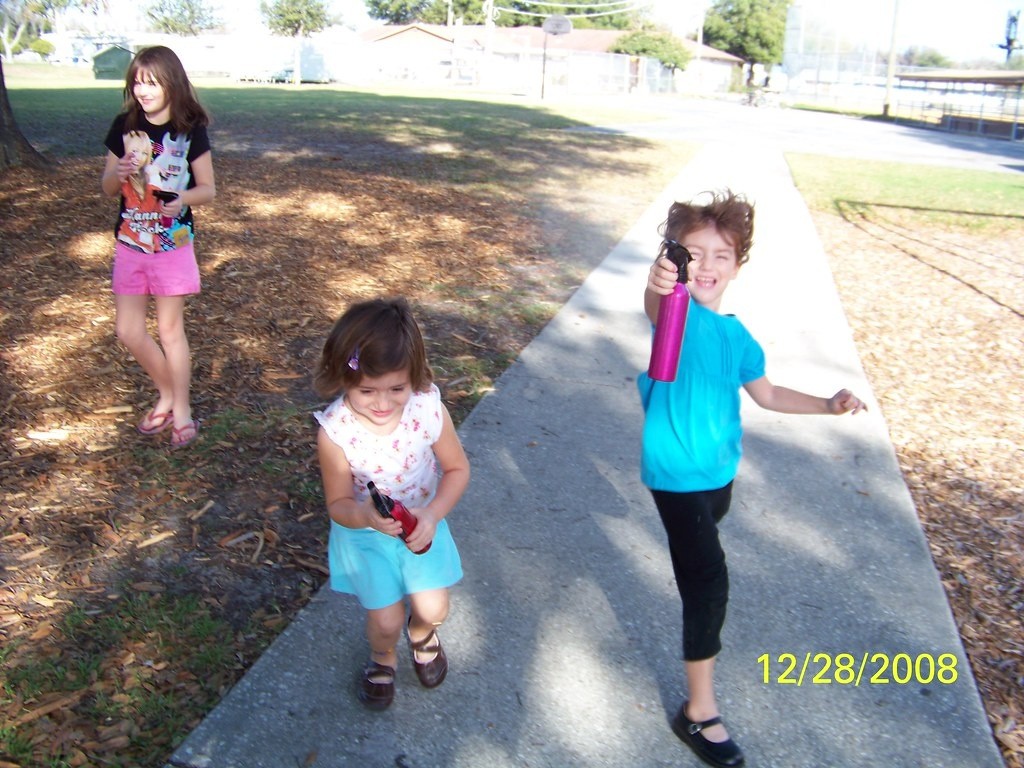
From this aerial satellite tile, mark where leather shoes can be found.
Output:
[407,615,448,689]
[671,701,743,768]
[357,659,394,708]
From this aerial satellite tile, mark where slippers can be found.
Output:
[170,421,199,449]
[137,405,173,434]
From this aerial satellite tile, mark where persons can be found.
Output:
[99,45,218,447]
[312,296,470,713]
[637,190,867,767]
